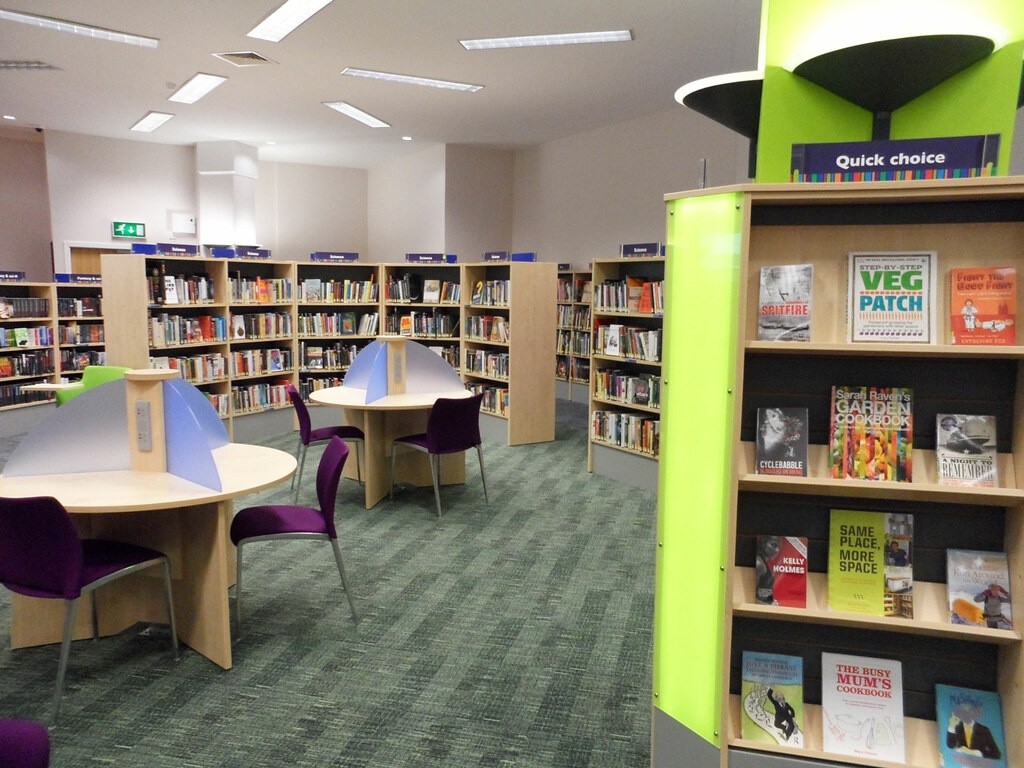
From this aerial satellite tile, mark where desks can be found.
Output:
[0,368,300,670]
[309,336,472,509]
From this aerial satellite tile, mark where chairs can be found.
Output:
[230,434,359,642]
[0,495,180,730]
[389,392,490,518]
[288,384,365,505]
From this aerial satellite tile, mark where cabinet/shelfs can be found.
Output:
[651,175,1024,767]
[0,254,665,494]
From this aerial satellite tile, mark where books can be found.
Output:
[939,407,996,486]
[947,549,1013,630]
[591,410,660,456]
[594,367,660,409]
[832,380,920,481]
[465,280,510,417]
[756,536,808,609]
[556,278,592,382]
[758,407,814,481]
[228,271,292,413]
[147,275,228,418]
[298,312,379,336]
[758,251,1018,346]
[742,651,803,748]
[595,274,664,315]
[299,342,363,403]
[827,510,909,621]
[298,274,379,303]
[935,683,1007,768]
[385,273,460,372]
[58,297,104,383]
[0,297,54,407]
[822,652,905,762]
[593,319,662,362]
